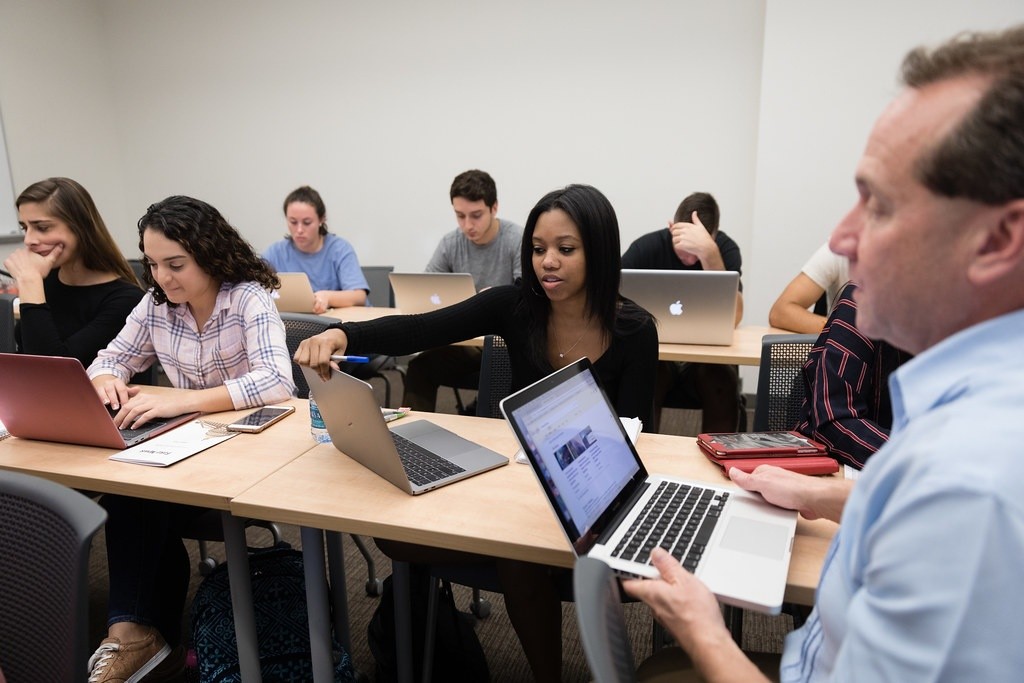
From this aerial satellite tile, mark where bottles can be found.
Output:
[308,389,331,442]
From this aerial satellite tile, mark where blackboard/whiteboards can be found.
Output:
[0,115,25,243]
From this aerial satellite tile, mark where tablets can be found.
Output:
[699,431,827,456]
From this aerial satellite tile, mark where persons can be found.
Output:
[795,279,914,471]
[5,177,154,385]
[769,241,854,335]
[401,170,523,414]
[257,186,371,313]
[86,196,300,683]
[620,192,747,437]
[292,183,660,683]
[622,25,1024,683]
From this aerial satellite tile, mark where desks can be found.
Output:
[0,385,841,683]
[329,307,814,367]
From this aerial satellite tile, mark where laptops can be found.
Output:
[266,272,334,314]
[388,271,485,340]
[301,361,509,496]
[619,269,740,346]
[0,351,201,450]
[497,355,798,614]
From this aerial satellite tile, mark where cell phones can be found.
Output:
[226,405,296,433]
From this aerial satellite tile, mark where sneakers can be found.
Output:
[87,625,172,683]
[149,644,185,683]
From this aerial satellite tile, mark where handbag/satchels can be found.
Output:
[368,574,491,683]
[185,549,350,683]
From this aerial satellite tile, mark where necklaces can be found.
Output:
[549,314,592,358]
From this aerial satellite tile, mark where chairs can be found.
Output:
[178,312,381,598]
[417,335,511,683]
[0,469,108,683]
[753,334,821,432]
[360,266,396,306]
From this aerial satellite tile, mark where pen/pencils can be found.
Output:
[330,355,369,363]
